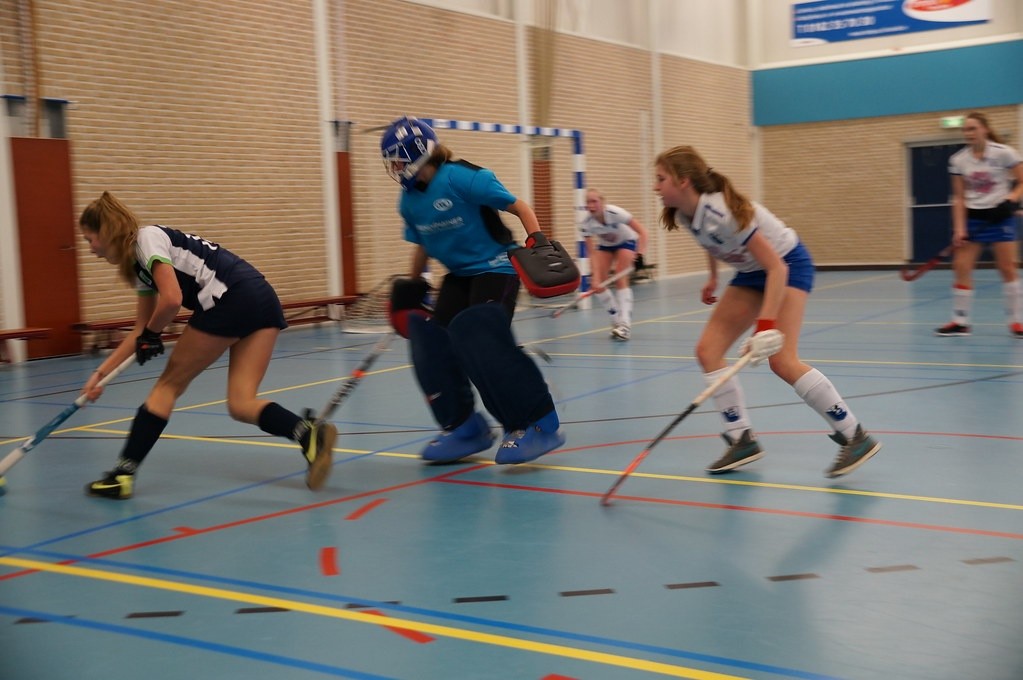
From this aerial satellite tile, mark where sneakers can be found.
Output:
[89,469,135,498]
[705,428,766,474]
[934,321,972,336]
[609,318,631,340]
[297,409,338,492]
[1009,329,1023,339]
[824,423,882,478]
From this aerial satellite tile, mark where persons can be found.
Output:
[652,146,882,481]
[579,188,650,339]
[79,191,340,493]
[933,111,1023,340]
[386,116,582,465]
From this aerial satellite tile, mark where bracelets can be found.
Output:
[93,369,107,377]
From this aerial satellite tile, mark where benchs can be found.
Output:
[0,327,52,363]
[71,294,361,354]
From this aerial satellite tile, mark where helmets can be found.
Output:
[379,113,439,193]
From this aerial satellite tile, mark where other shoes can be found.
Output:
[422,414,495,462]
[495,426,567,466]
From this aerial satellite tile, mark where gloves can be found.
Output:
[990,200,1015,226]
[634,254,644,271]
[136,325,164,366]
[739,320,782,365]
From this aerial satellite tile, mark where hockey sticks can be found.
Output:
[315,326,396,420]
[599,349,754,508]
[549,262,658,319]
[0,352,137,498]
[900,199,1020,282]
